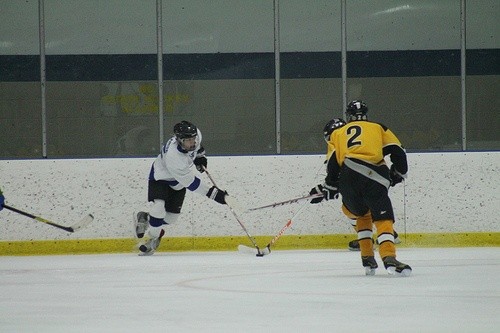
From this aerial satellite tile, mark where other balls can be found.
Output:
[256,254,264,257]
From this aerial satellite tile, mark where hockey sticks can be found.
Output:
[224,192,325,217]
[236,160,326,258]
[202,165,265,259]
[4,205,95,233]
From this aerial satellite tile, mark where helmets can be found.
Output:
[346,100,368,116]
[174,120,198,150]
[324,118,347,143]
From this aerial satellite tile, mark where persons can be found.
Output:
[308,118,402,251]
[322,98,413,278]
[134,120,228,255]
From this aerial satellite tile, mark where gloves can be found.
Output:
[308,184,326,204]
[206,186,229,204]
[323,185,339,201]
[193,156,207,173]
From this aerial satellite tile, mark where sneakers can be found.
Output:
[383,256,412,276]
[362,258,378,276]
[376,229,398,243]
[138,228,165,256]
[133,210,149,239]
[349,239,376,250]
